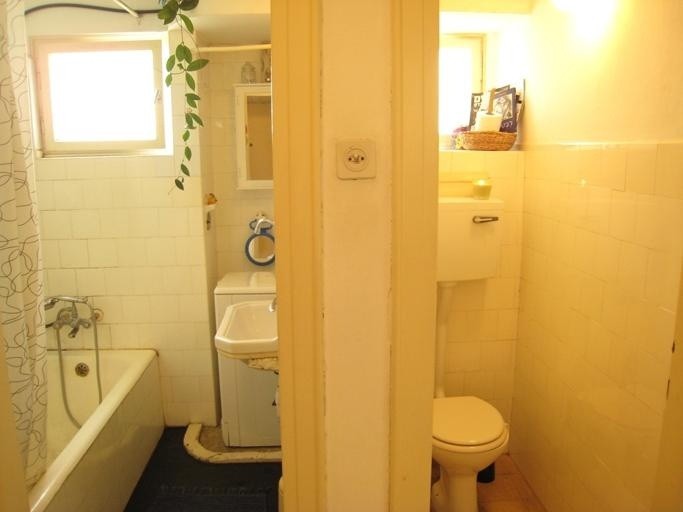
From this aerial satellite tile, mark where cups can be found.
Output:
[472,176,491,200]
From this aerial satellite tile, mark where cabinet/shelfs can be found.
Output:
[233,82,275,190]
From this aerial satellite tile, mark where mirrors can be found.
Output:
[243,220,275,268]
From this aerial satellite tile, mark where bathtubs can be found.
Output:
[27,349,165,512]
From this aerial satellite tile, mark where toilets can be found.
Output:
[432,395,510,512]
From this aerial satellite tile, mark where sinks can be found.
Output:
[213,271,276,333]
[214,299,277,354]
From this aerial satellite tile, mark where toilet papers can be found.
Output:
[474,110,502,132]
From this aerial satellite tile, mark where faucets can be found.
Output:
[254,218,273,234]
